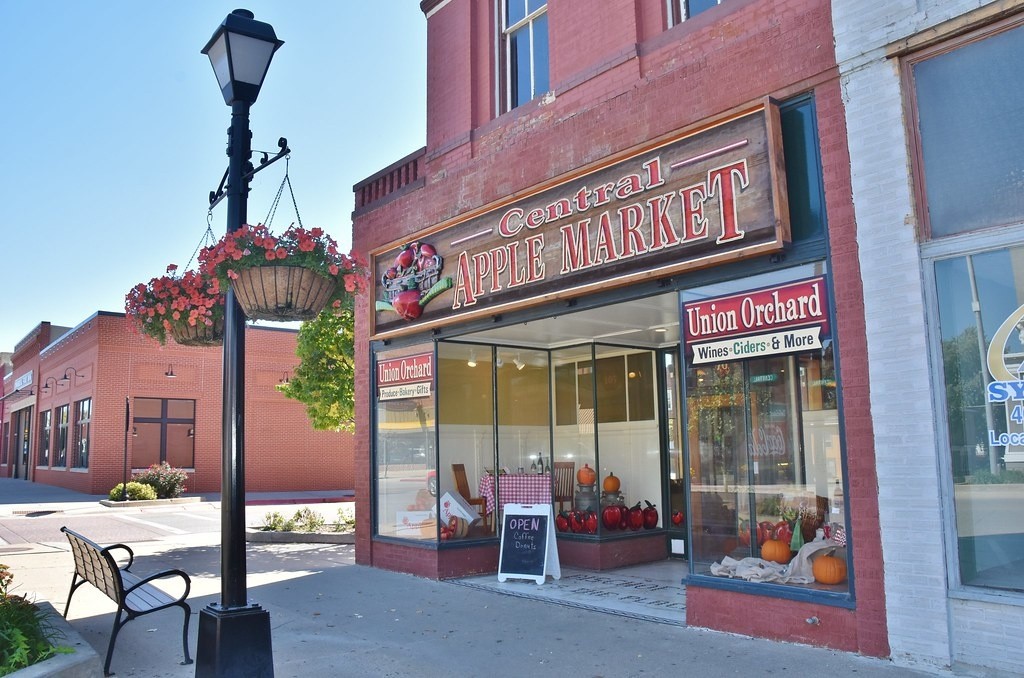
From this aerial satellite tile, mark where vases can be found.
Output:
[159,308,222,344]
[228,267,335,321]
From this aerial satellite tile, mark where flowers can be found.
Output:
[196,226,371,294]
[123,263,227,346]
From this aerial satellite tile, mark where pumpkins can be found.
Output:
[761,535,792,564]
[577,463,596,485]
[812,549,847,584]
[603,472,620,493]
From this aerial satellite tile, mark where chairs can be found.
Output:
[451,463,491,533]
[554,461,576,517]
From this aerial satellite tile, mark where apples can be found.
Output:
[407,497,432,511]
[440,519,456,540]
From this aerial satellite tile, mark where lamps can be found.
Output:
[279,372,288,382]
[187,429,194,437]
[28,385,47,395]
[497,350,503,367]
[133,427,138,436]
[42,377,63,389]
[513,353,525,370]
[16,389,35,395]
[165,364,176,377]
[467,350,479,367]
[60,367,83,380]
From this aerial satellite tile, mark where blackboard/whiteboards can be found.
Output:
[500,514,548,576]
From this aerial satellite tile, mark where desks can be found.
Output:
[477,474,557,521]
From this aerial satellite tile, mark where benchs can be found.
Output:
[58,525,193,678]
